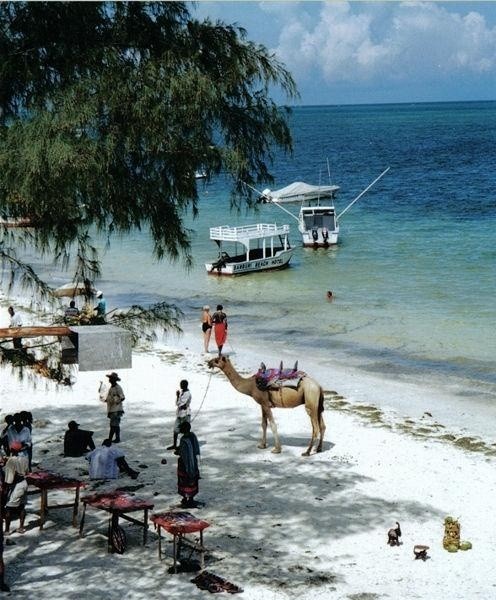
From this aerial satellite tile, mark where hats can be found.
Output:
[95,290,104,298]
[68,421,80,428]
[106,372,121,381]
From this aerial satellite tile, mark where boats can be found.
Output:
[205,219,300,279]
[257,177,341,204]
[1,200,90,228]
[235,160,394,250]
[191,169,206,179]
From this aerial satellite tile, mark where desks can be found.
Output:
[78,491,154,561]
[149,511,211,575]
[17,470,85,535]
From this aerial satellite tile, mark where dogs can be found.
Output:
[389,521,401,546]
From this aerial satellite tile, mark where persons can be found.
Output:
[0,410,32,597]
[165,378,193,456]
[199,304,214,354]
[97,372,124,444]
[62,420,95,458]
[63,300,81,348]
[322,290,335,301]
[91,289,107,321]
[172,420,201,509]
[209,304,229,359]
[6,306,25,350]
[83,438,138,480]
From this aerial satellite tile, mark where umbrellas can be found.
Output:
[46,280,98,308]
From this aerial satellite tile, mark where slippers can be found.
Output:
[190,570,244,595]
